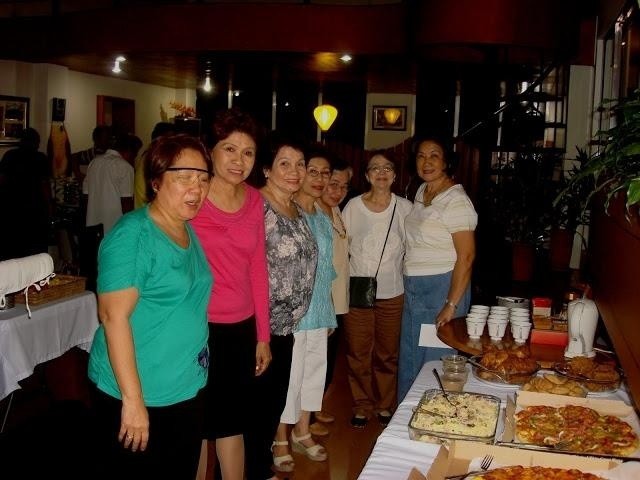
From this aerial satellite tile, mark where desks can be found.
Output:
[0,289,101,433]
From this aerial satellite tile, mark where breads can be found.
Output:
[519,373,586,398]
[475,340,537,384]
[566,356,620,392]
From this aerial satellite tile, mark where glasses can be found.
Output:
[367,165,395,174]
[166,167,215,185]
[330,181,352,192]
[306,167,332,178]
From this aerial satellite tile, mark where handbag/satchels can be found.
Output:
[77,223,104,251]
[347,276,377,309]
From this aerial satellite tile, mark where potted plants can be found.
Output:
[492,145,549,281]
[550,149,594,270]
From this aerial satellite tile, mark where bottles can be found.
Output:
[564,292,579,304]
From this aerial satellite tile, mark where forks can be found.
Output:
[445,454,494,480]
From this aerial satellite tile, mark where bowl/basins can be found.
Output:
[440,353,469,392]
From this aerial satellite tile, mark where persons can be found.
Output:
[1,110,477,480]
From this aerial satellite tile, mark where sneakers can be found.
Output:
[375,411,391,426]
[350,414,368,429]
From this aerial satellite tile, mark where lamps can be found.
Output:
[203,63,213,94]
[112,57,122,74]
[384,107,402,124]
[313,104,339,132]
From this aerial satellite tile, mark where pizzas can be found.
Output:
[512,403,640,457]
[469,464,610,480]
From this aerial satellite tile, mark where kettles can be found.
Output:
[564,299,599,357]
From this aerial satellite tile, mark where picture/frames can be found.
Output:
[372,104,407,131]
[0,95,30,146]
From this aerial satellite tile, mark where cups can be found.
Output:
[465,304,532,344]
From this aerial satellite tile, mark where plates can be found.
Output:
[469,356,541,386]
[551,362,621,395]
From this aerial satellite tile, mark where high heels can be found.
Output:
[270,440,295,472]
[290,429,328,462]
[307,422,330,437]
[315,407,335,424]
